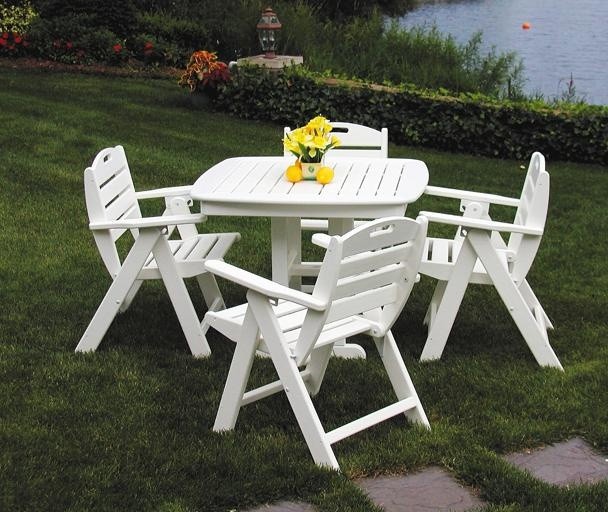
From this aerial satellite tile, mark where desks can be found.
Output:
[191,157,429,290]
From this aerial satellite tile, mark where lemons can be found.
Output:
[317,167,333,183]
[295,159,302,169]
[286,166,303,181]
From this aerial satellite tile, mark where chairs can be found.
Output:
[75,145,242,360]
[283,121,389,292]
[416,151,566,374]
[203,215,432,474]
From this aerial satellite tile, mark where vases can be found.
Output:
[301,163,322,180]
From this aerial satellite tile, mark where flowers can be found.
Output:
[281,116,342,163]
